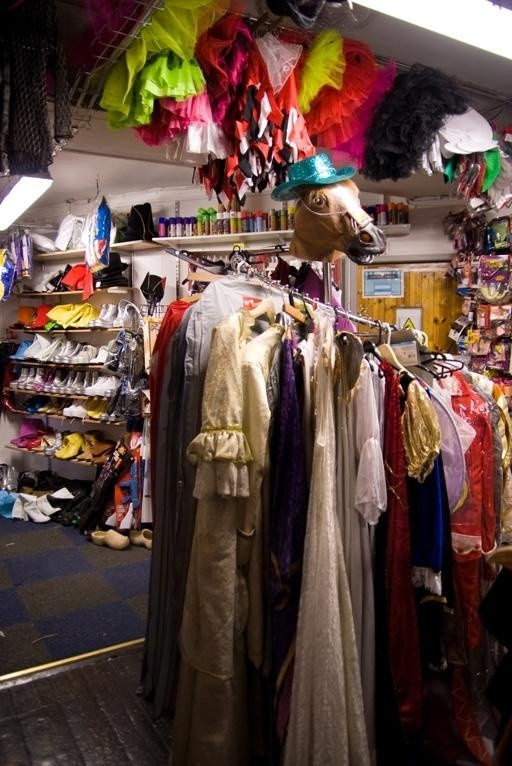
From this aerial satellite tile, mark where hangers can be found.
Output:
[226,269,464,384]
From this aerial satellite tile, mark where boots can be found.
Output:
[9,418,115,462]
[8,333,119,365]
[27,394,125,422]
[0,461,20,490]
[46,438,135,536]
[9,367,122,397]
[8,299,142,328]
[11,487,75,523]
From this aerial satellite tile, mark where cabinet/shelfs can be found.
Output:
[14,229,295,529]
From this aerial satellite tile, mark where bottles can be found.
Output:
[158,202,410,238]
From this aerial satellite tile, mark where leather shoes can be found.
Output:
[91,529,130,550]
[129,528,152,549]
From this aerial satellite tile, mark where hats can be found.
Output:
[270,153,358,200]
[95,251,131,288]
